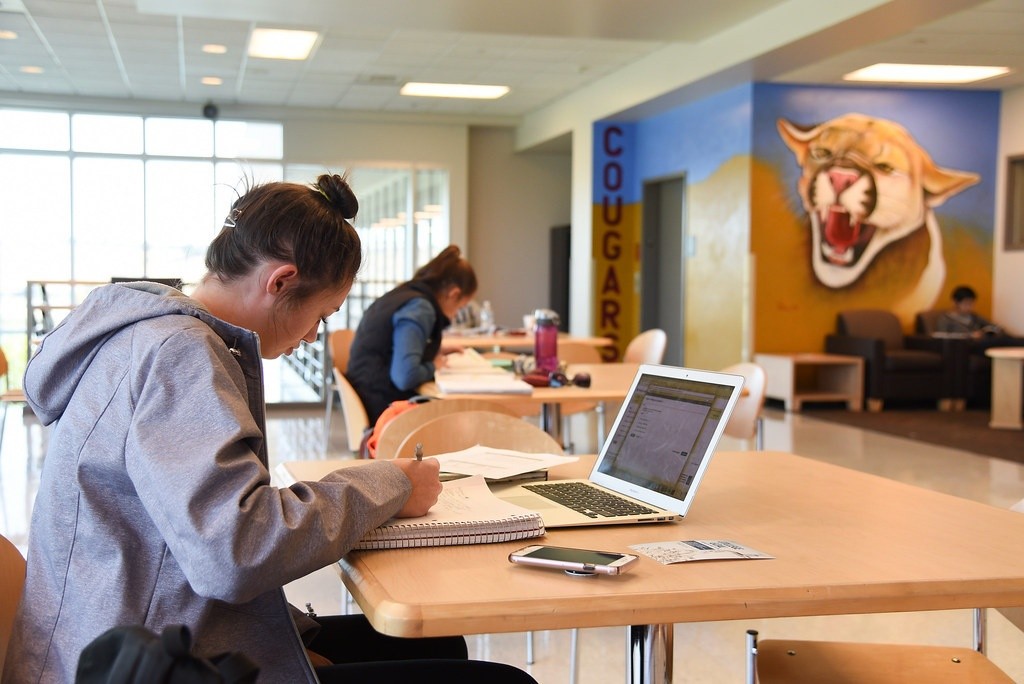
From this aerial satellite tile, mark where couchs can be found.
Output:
[827,306,942,412]
[905,307,1024,407]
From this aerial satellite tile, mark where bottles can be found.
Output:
[534,309,559,373]
[480,300,494,328]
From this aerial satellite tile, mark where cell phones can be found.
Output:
[508,544,640,576]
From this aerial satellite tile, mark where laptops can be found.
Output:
[488,362,746,528]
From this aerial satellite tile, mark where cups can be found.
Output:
[523,315,534,333]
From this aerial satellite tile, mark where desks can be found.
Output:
[410,358,676,451]
[753,349,864,412]
[987,345,1024,430]
[286,448,1023,684]
[440,334,616,364]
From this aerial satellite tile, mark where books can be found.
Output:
[351,471,545,549]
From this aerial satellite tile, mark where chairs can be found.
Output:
[623,329,668,368]
[710,361,766,448]
[744,629,1014,683]
[0,536,26,684]
[332,366,372,457]
[376,396,519,459]
[0,350,47,452]
[394,412,563,458]
[326,330,358,368]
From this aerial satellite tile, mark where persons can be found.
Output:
[0,174,538,684]
[939,284,990,338]
[348,246,479,428]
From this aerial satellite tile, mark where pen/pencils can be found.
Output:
[416,443,423,460]
